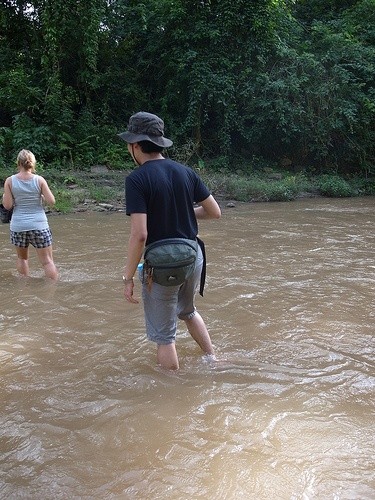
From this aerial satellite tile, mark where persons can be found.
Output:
[2,149,58,279]
[117,112,221,369]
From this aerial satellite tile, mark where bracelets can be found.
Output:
[122,276,133,282]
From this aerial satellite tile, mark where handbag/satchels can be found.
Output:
[142,237,198,286]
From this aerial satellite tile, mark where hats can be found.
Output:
[116,112,173,147]
